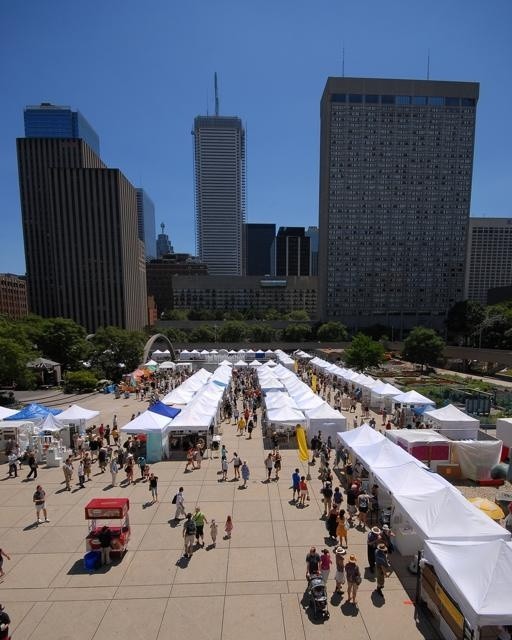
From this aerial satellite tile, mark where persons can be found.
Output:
[0,605,11,640]
[8,355,434,605]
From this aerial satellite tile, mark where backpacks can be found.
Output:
[171,493,181,504]
[186,522,195,535]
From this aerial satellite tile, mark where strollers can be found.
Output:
[307,571,330,621]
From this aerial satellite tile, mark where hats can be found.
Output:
[371,523,392,551]
[335,546,358,563]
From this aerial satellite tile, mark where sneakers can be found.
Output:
[37,519,50,524]
